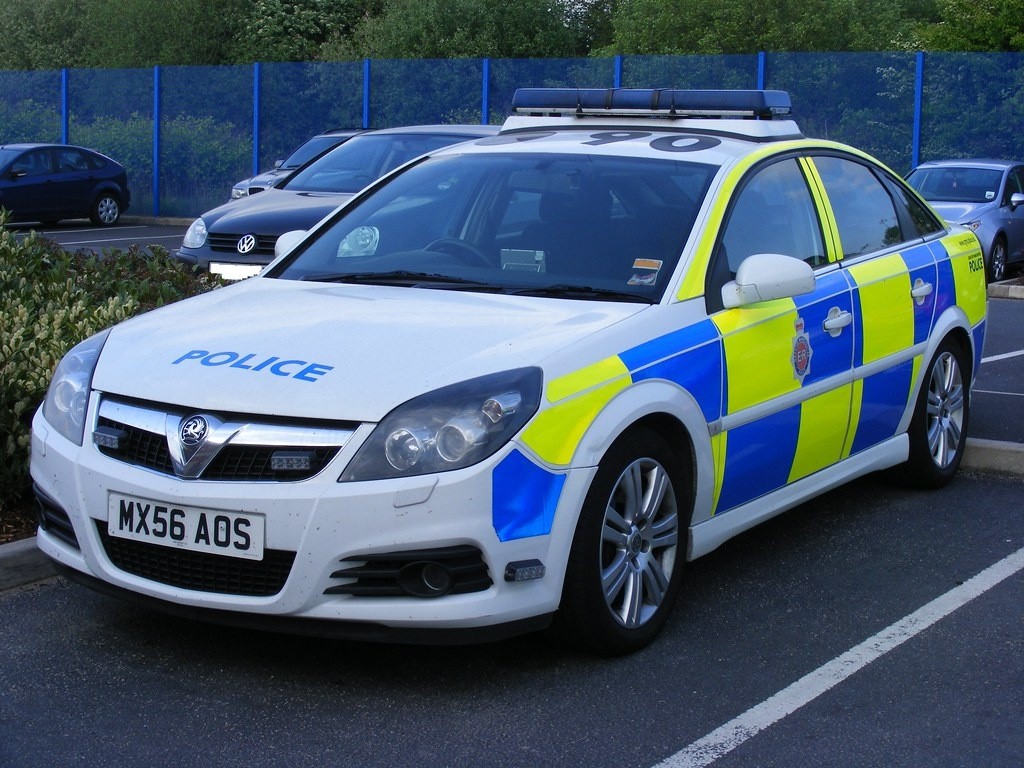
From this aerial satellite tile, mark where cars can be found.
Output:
[0,141,132,228]
[894,157,1024,281]
[24,87,991,655]
[172,124,626,299]
[228,123,412,211]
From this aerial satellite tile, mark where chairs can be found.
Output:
[520,193,589,278]
[936,177,964,198]
[726,192,796,273]
